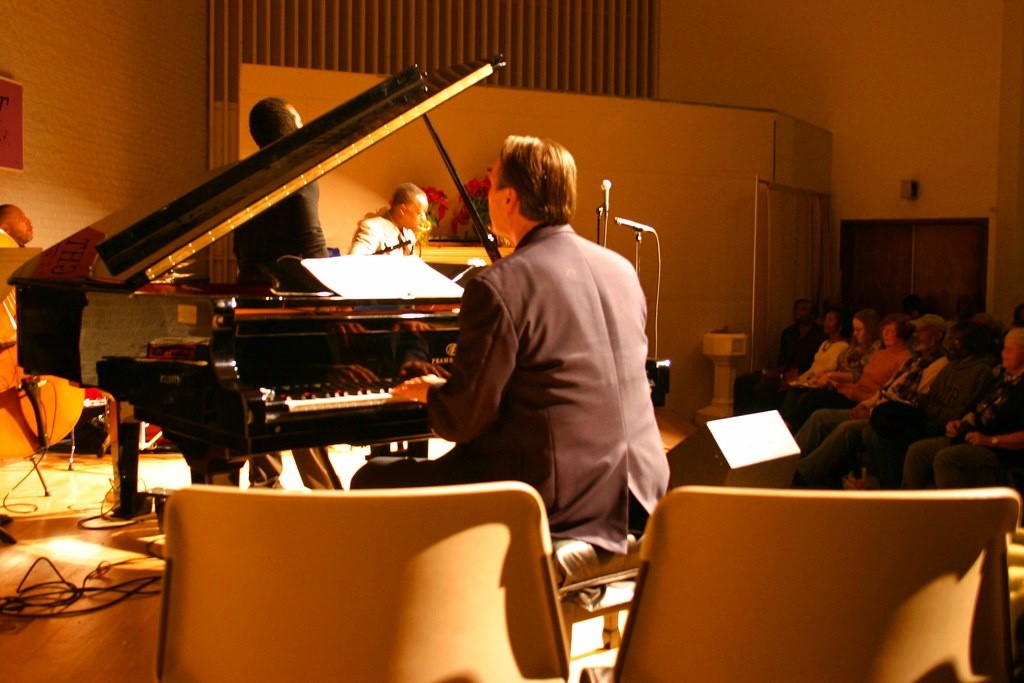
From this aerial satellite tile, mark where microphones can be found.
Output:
[615,217,654,233]
[601,179,612,219]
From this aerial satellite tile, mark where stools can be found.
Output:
[68,387,107,470]
[551,534,639,650]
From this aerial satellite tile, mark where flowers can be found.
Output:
[421,185,448,230]
[455,167,492,227]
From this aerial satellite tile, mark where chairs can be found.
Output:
[579,485,1021,683]
[151,479,571,683]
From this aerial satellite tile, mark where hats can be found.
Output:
[907,314,947,333]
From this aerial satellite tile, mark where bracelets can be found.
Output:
[992,436,998,447]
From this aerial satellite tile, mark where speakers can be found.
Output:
[666,409,801,492]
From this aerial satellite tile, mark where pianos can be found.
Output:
[7,56,673,487]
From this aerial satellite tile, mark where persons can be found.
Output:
[734,300,1024,529]
[349,135,671,555]
[248,97,342,489]
[349,183,428,255]
[0,204,33,248]
